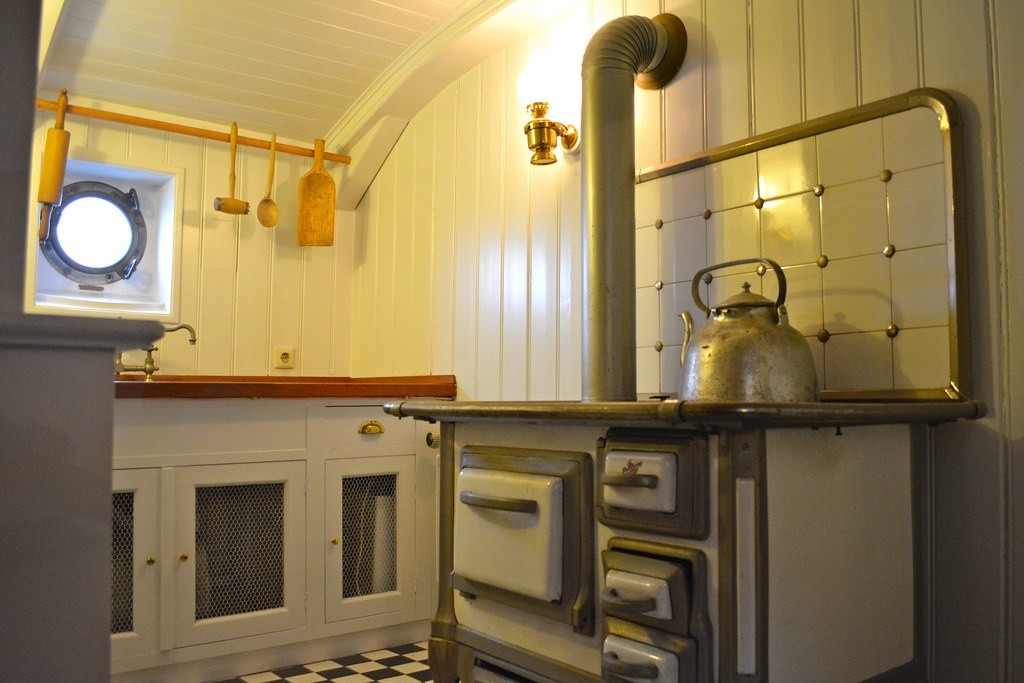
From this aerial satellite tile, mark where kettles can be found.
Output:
[674,255,820,406]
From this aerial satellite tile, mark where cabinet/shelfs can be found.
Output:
[111,398,457,683]
[0,0,165,683]
